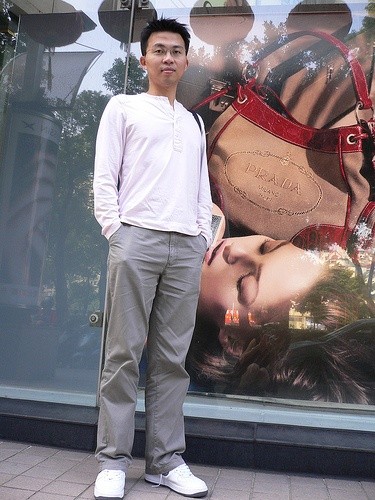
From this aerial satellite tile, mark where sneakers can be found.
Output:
[93,469,125,500]
[145,463,208,497]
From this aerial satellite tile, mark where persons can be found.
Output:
[185,200,374,408]
[91,13,212,497]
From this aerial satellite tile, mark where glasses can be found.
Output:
[145,47,187,58]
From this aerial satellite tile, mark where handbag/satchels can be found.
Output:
[186,29,374,267]
